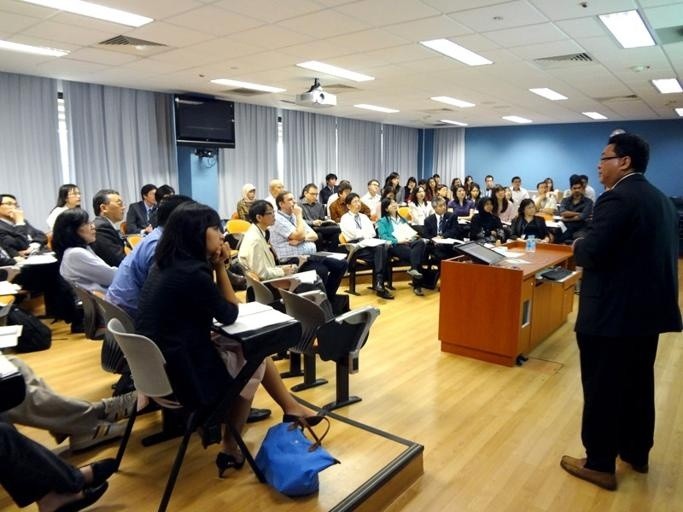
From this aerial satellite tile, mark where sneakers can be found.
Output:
[101,389,137,422]
[407,269,423,280]
[69,422,129,452]
[413,287,424,295]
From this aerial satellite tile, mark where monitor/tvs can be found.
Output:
[452,241,505,267]
[172,93,237,150]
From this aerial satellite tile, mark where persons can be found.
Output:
[267,191,348,299]
[1,352,148,511]
[560,130,683,491]
[296,184,327,226]
[263,180,286,212]
[238,201,333,319]
[1,194,84,333]
[49,184,198,397]
[237,183,257,222]
[319,173,595,299]
[138,203,327,478]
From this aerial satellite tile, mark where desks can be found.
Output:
[437,240,582,368]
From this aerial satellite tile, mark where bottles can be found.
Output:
[469,207,475,217]
[448,205,454,212]
[525,235,536,252]
[556,201,561,210]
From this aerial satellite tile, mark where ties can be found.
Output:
[438,216,444,232]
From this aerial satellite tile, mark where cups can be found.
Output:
[29,241,41,252]
[495,239,501,247]
[463,238,469,243]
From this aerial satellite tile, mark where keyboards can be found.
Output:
[542,267,572,281]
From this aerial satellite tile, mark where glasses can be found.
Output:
[600,157,621,163]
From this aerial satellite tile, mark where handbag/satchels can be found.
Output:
[254,415,341,497]
[7,304,52,353]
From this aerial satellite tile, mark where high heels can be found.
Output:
[216,450,246,478]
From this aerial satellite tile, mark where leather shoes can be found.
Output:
[560,455,617,491]
[376,289,394,299]
[52,481,108,511]
[77,457,116,487]
[283,409,328,426]
[246,408,271,423]
[374,281,384,291]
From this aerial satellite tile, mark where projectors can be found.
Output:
[295,92,336,109]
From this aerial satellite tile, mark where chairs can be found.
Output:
[2,219,381,511]
[221,189,564,297]
